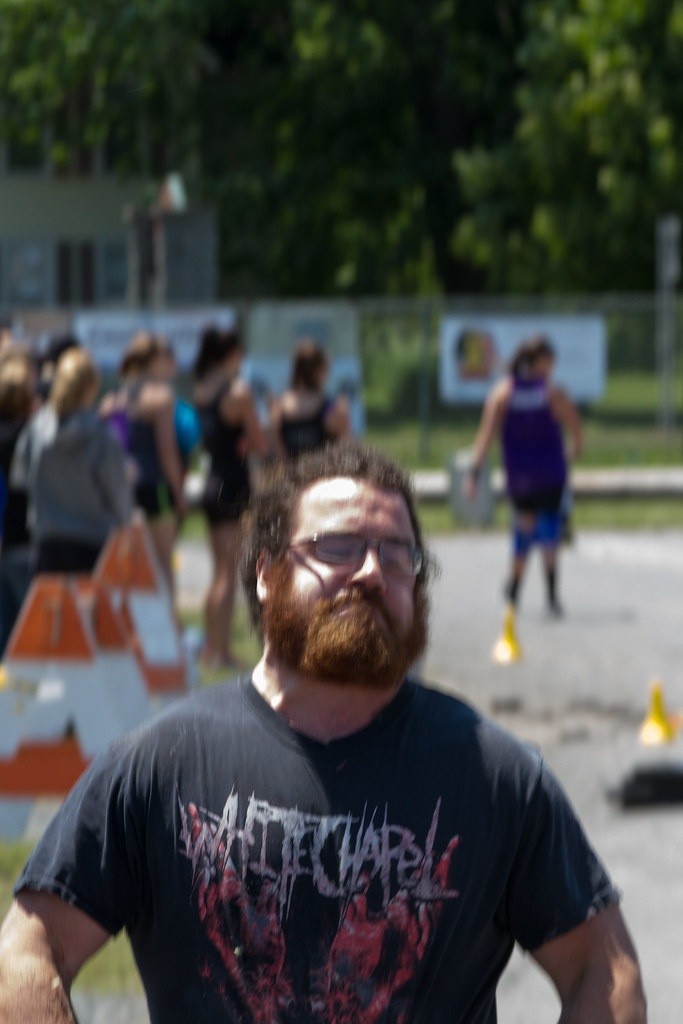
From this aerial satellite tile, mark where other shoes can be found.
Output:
[200,650,234,670]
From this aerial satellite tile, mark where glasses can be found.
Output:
[282,533,422,575]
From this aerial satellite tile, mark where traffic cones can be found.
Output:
[491,605,522,663]
[1,507,198,843]
[637,679,676,745]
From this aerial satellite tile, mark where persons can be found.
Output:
[459,331,584,621]
[0,442,653,1024]
[1,314,350,672]
